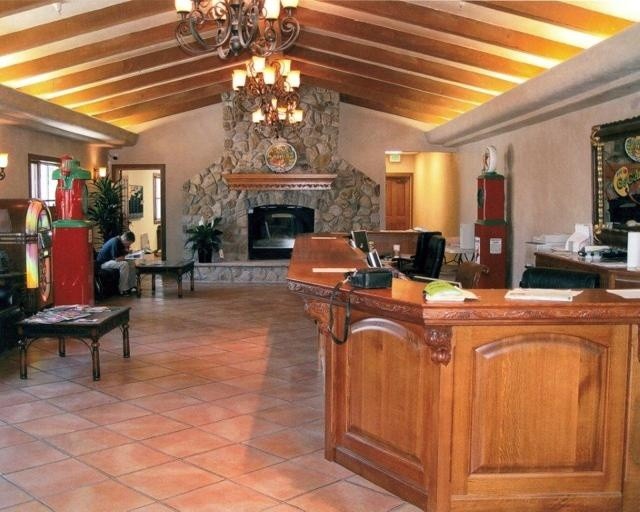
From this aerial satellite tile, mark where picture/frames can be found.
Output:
[590,115,640,247]
[128,185,144,218]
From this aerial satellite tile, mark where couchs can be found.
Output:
[94,249,119,301]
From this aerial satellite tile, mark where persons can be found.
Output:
[96,231,142,296]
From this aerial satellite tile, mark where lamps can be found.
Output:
[93,167,106,184]
[174,0,305,128]
[0,153,9,181]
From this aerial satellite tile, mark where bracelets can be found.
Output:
[125,250,144,260]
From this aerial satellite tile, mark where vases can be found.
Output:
[198,248,213,263]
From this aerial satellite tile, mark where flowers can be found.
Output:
[184,216,225,260]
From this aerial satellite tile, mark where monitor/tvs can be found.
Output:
[351,229,369,253]
[367,248,383,268]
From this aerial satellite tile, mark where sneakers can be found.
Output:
[122,287,137,297]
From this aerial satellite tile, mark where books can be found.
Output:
[351,229,369,251]
[25,307,92,323]
[424,280,477,302]
[503,285,582,302]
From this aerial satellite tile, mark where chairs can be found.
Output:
[391,231,615,289]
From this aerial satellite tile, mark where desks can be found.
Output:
[134,260,195,298]
[16,304,132,381]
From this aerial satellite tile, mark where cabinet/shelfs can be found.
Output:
[534,250,640,289]
[0,249,26,352]
